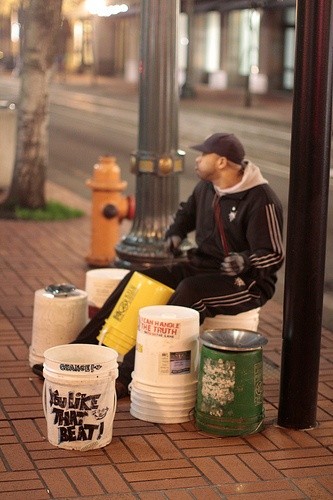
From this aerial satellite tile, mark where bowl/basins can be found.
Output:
[43,284,76,296]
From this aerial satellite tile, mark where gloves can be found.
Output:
[165,235,183,257]
[221,252,244,276]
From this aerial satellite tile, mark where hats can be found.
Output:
[190,132,245,165]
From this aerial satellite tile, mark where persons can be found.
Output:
[32,130,286,398]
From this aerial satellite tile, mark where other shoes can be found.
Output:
[115,379,129,399]
[31,363,45,381]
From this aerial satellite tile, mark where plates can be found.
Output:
[199,328,269,352]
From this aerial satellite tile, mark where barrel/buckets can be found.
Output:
[192,345,266,436]
[96,269,175,355]
[129,306,200,424]
[42,343,119,452]
[28,288,89,367]
[86,267,130,323]
